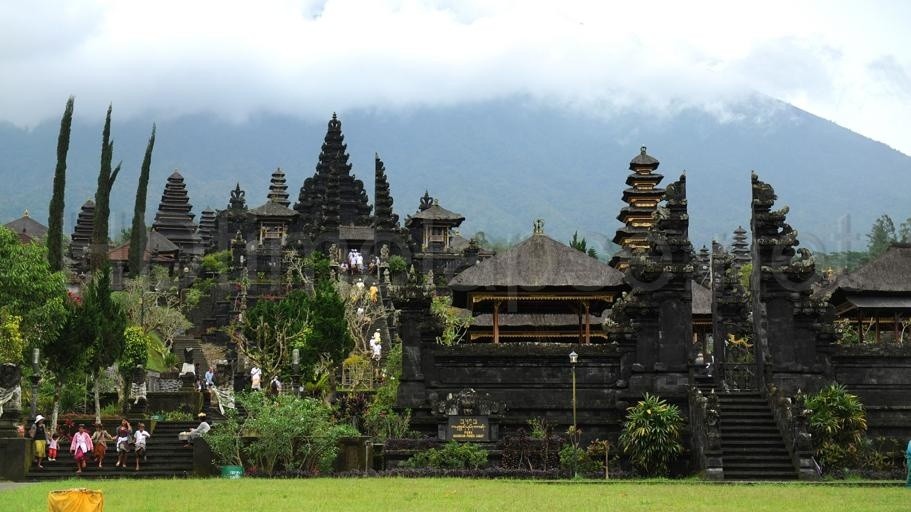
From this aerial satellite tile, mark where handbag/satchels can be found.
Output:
[93,439,98,445]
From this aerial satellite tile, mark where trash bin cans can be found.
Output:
[373,443,384,469]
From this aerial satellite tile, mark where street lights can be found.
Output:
[569,350,580,432]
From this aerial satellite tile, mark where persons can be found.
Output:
[340,248,384,365]
[383,268,391,283]
[194,362,216,404]
[15,413,151,471]
[269,376,283,397]
[249,364,263,391]
[184,416,211,447]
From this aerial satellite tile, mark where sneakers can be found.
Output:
[35,457,56,468]
[184,444,192,448]
[136,456,147,470]
[76,458,103,473]
[116,461,127,467]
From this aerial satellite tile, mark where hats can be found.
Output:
[35,415,45,424]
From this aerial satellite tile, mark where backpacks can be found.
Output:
[272,379,277,389]
